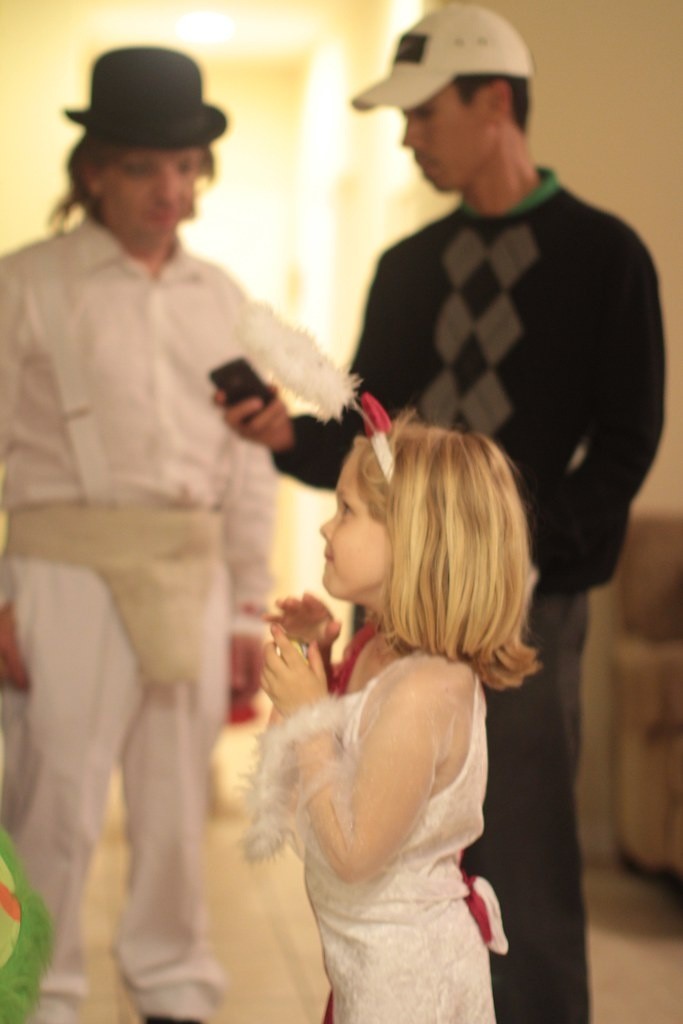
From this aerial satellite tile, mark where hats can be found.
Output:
[65,46,227,147]
[352,4,532,112]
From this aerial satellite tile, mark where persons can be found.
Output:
[211,0,665,1024]
[0,46,280,1024]
[246,394,537,1024]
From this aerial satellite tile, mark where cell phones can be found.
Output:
[210,358,273,426]
